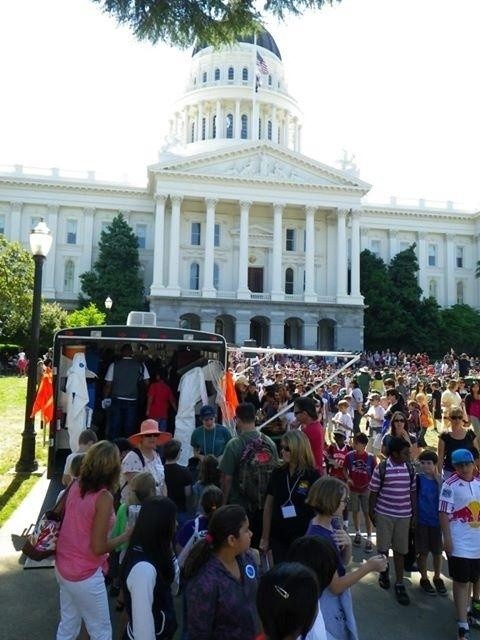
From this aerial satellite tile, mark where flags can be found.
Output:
[256,50,270,76]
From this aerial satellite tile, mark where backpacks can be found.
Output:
[237,432,278,503]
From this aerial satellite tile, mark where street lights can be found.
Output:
[102,295,115,326]
[8,219,55,481]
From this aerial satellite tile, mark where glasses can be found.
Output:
[340,497,350,505]
[145,433,160,437]
[294,410,303,415]
[450,415,464,419]
[279,444,291,452]
[394,418,406,422]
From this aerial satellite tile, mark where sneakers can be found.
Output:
[352,535,479,639]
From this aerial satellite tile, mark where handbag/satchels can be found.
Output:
[21,511,63,562]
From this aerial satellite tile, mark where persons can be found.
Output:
[1,350,29,378]
[37,341,480,638]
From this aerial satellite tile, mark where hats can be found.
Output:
[365,393,380,400]
[349,433,367,444]
[200,405,218,419]
[127,419,172,446]
[334,429,346,440]
[452,448,474,465]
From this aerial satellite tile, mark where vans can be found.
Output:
[18,311,232,571]
